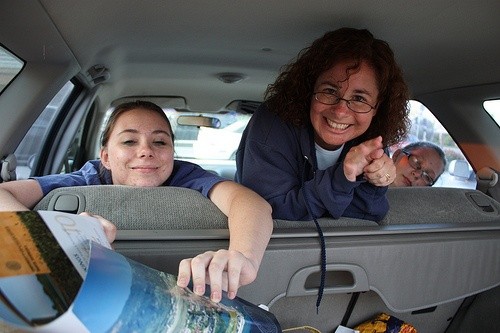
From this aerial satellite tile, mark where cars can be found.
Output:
[1,85,481,186]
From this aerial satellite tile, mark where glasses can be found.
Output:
[314,83,382,114]
[402,149,435,187]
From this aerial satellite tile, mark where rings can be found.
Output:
[386,173,391,181]
[354,148,358,152]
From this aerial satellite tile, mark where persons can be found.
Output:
[389,141,448,190]
[0,99,274,301]
[232,26,413,223]
